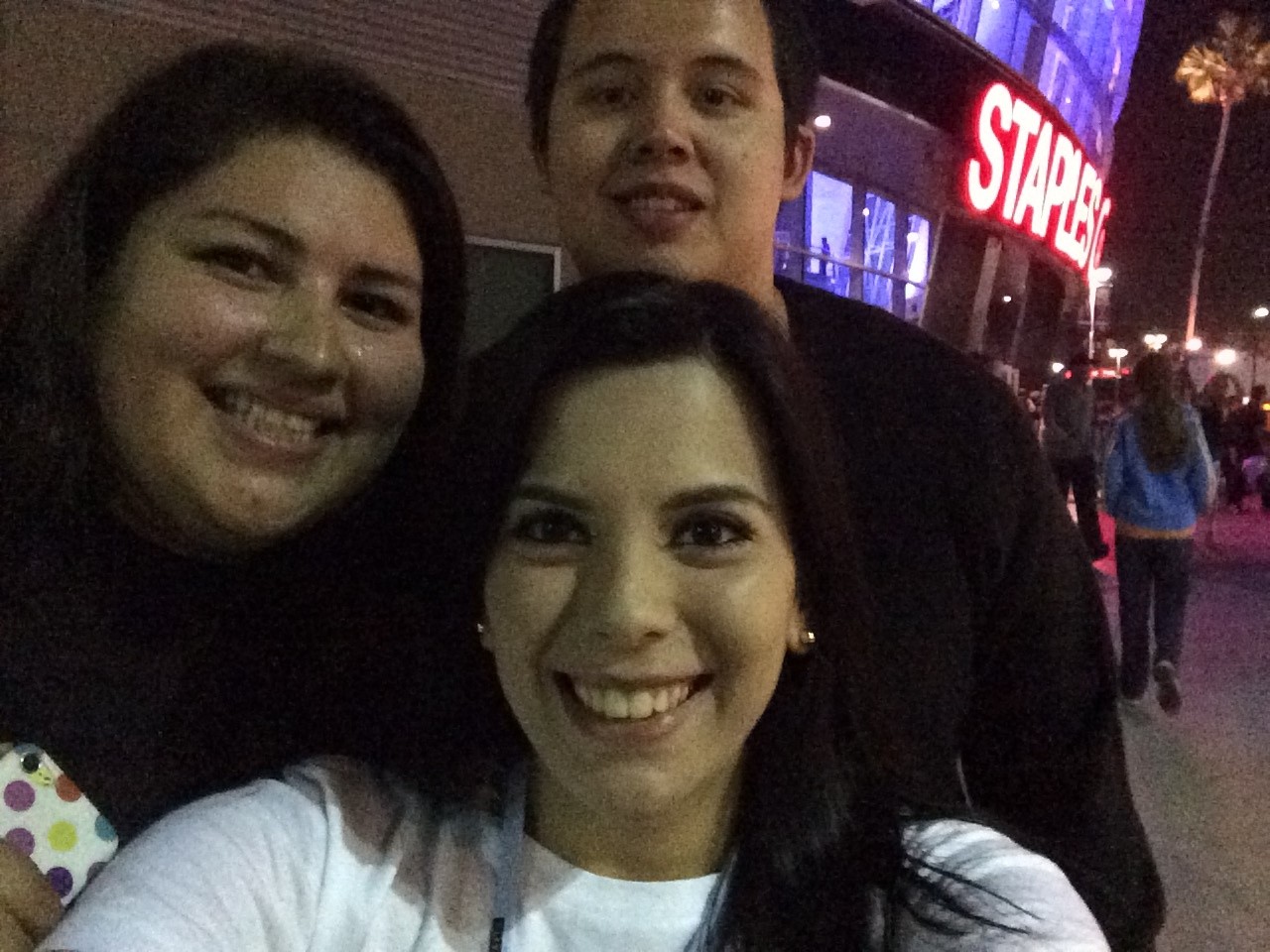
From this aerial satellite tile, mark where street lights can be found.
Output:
[1146,331,1167,356]
[1215,347,1235,378]
[1107,348,1129,380]
[1250,304,1268,402]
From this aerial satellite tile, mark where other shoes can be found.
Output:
[1091,544,1108,561]
[1153,659,1183,716]
[1124,694,1144,705]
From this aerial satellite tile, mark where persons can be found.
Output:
[0,36,470,952]
[818,237,830,275]
[517,0,1164,952]
[32,267,1112,952]
[966,348,1270,715]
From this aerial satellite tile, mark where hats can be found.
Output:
[1069,352,1094,365]
